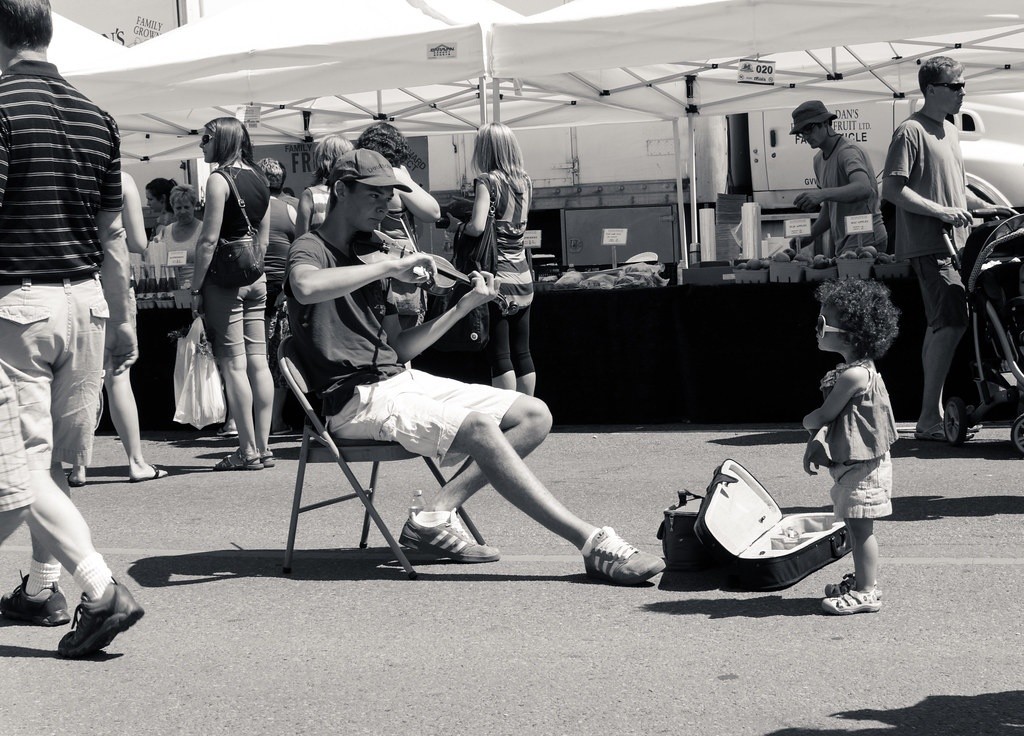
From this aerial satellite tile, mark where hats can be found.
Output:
[330,148,413,193]
[789,101,836,135]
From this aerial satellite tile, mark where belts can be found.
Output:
[0,269,101,287]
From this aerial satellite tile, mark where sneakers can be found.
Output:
[58,576,144,657]
[0,569,72,627]
[399,508,501,563]
[584,526,666,587]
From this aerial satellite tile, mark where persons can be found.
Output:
[66,170,168,487]
[132,177,204,289]
[883,57,1019,441]
[788,101,889,258]
[286,148,668,584]
[253,134,354,437]
[444,122,537,396]
[187,116,278,470]
[0,0,146,660]
[803,277,901,614]
[358,121,441,370]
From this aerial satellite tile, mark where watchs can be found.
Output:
[190,289,202,295]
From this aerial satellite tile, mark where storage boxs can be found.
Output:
[735,258,911,283]
[173,290,192,308]
[136,300,155,309]
[155,300,174,308]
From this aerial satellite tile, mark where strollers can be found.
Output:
[943,207,1024,458]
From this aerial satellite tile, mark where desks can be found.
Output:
[95,281,930,436]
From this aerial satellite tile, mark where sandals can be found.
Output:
[260,447,277,467]
[822,589,882,614]
[824,572,879,597]
[214,448,264,469]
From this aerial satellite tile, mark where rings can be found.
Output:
[193,309,198,312]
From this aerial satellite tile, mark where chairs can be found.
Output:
[279,336,486,580]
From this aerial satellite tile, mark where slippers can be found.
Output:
[67,471,87,487]
[966,423,983,433]
[215,427,238,436]
[914,422,974,442]
[129,464,169,483]
[272,423,293,434]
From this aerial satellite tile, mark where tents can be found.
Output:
[47,0,1024,186]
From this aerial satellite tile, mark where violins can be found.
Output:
[351,229,520,316]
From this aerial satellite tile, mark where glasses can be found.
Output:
[933,82,967,92]
[202,133,217,145]
[797,123,815,138]
[818,314,848,338]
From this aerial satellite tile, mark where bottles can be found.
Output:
[408,490,427,516]
[131,264,178,299]
[567,264,575,272]
[677,260,686,285]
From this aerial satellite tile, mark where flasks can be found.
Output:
[690,243,701,266]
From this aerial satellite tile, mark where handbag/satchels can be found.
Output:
[267,309,290,386]
[206,170,263,287]
[172,316,227,430]
[365,222,429,315]
[455,215,499,278]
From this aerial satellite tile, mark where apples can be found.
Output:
[736,245,902,270]
